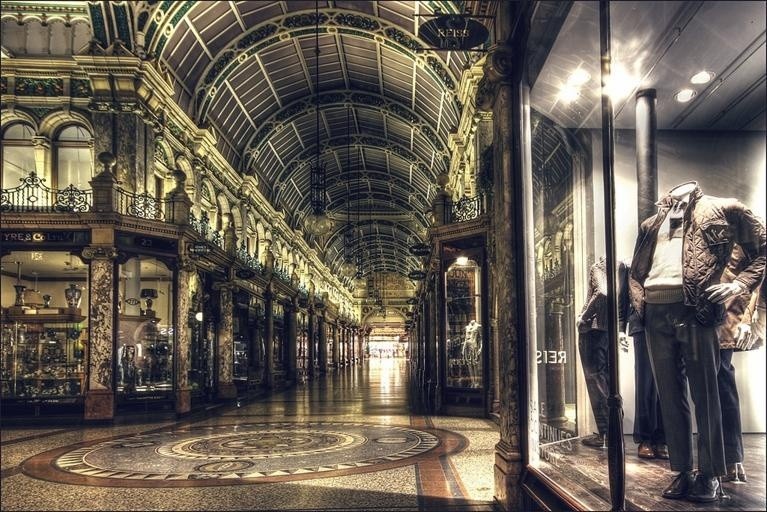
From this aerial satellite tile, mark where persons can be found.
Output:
[717,243,766,483]
[617,254,670,460]
[462,320,483,389]
[576,255,619,448]
[628,180,767,503]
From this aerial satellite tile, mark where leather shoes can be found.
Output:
[721,463,746,483]
[637,443,669,459]
[582,435,604,447]
[662,470,696,499]
[687,473,720,503]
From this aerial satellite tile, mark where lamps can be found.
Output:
[306,0,394,325]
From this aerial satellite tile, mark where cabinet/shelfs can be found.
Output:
[0,309,87,399]
